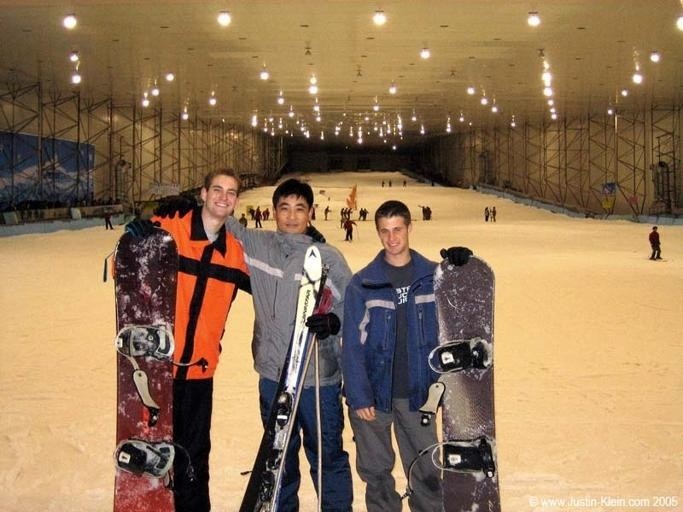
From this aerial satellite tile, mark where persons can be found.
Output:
[337,201,441,512]
[325,207,332,222]
[389,179,393,189]
[483,206,497,222]
[106,210,115,231]
[219,178,353,512]
[649,224,663,260]
[103,167,252,512]
[381,179,385,188]
[422,206,431,220]
[340,207,369,241]
[402,180,406,191]
[237,206,271,228]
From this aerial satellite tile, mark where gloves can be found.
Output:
[305,225,326,243]
[440,246,473,266]
[124,217,161,240]
[305,312,340,341]
[153,191,198,220]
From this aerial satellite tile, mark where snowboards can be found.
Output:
[242,246,329,512]
[112,227,175,511]
[433,255,500,512]
